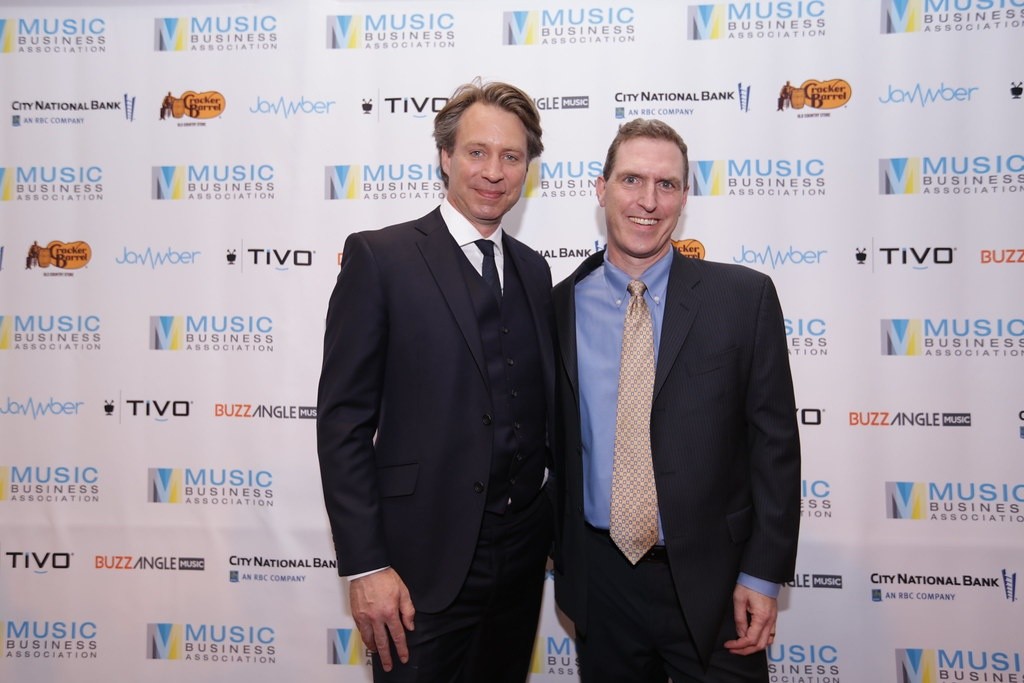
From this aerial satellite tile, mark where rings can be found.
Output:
[770,633,775,636]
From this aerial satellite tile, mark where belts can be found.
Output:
[585,523,667,561]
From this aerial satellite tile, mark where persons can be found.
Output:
[317,83,554,683]
[554,120,801,683]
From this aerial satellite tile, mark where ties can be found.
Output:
[474,239,503,308]
[610,278,659,565]
